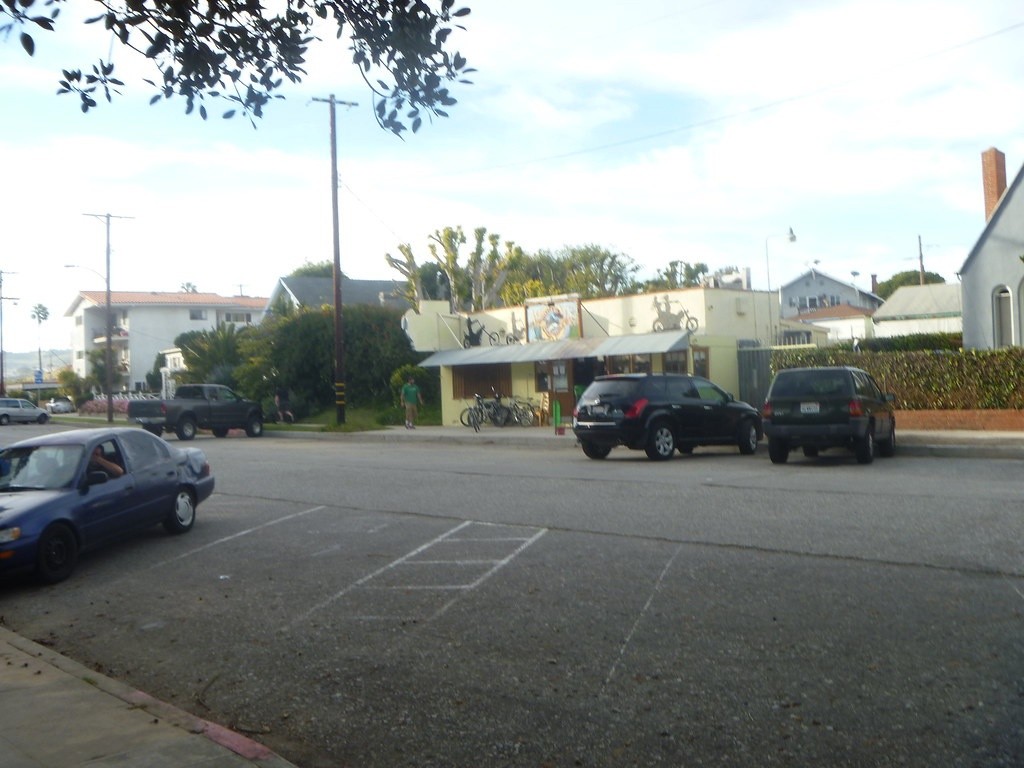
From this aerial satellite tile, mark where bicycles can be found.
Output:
[460,386,548,433]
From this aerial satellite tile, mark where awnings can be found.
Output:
[416,329,689,367]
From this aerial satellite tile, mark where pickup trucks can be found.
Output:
[128,384,263,441]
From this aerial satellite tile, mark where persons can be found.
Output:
[87,445,124,477]
[400,375,424,428]
[274,385,294,424]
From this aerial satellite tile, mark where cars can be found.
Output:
[45,397,74,414]
[0,427,214,583]
[761,367,896,464]
[572,371,764,460]
[0,398,50,426]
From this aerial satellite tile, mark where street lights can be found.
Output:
[0,298,20,397]
[766,225,797,345]
[64,265,114,423]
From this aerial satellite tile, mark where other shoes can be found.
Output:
[409,425,415,429]
[405,424,410,430]
